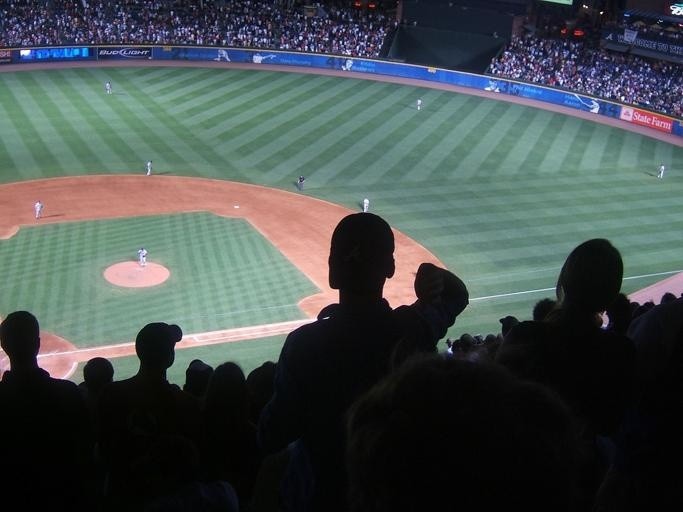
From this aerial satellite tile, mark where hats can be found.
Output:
[186,359,213,385]
[628,299,683,377]
[329,214,394,289]
[136,322,182,353]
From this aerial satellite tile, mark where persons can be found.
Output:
[363,196,370,212]
[298,176,304,189]
[658,160,665,180]
[1,311,275,511]
[34,201,43,219]
[488,22,683,118]
[256,213,681,510]
[2,0,395,58]
[146,160,152,177]
